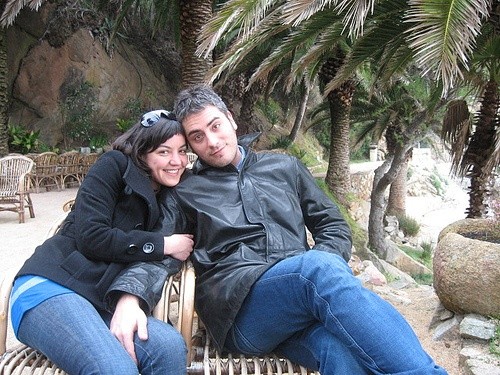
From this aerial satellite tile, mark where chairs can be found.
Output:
[0,151,321,375]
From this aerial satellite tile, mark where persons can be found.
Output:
[11,110,198,375]
[103,86,447,375]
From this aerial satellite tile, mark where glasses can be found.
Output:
[138,109,177,131]
